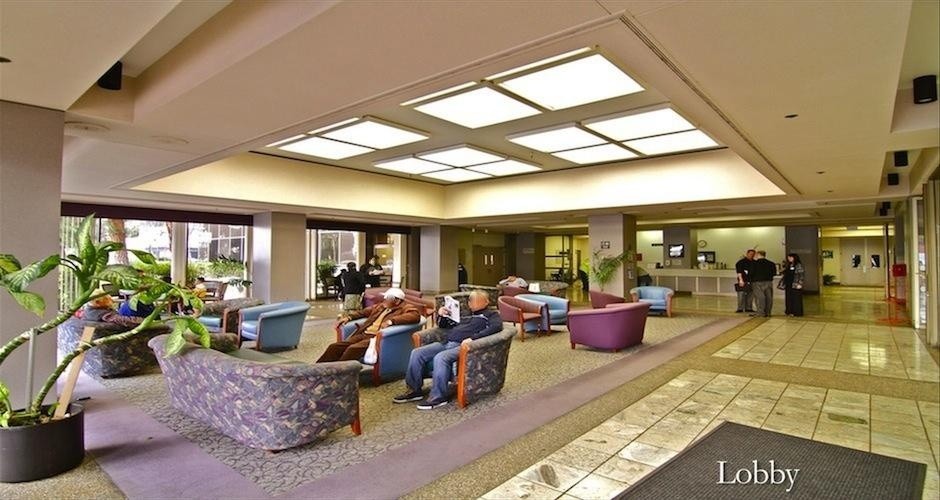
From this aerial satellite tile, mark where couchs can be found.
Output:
[148,332,361,454]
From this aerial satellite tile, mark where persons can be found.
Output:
[344,262,365,309]
[195,278,206,291]
[392,289,503,410]
[783,253,804,318]
[750,251,776,317]
[735,250,757,313]
[316,287,420,362]
[366,257,385,287]
[499,273,528,289]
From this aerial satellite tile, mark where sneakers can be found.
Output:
[417,396,448,409]
[392,388,424,403]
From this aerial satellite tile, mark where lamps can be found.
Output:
[888,173,899,185]
[913,75,938,104]
[879,202,890,216]
[894,151,908,167]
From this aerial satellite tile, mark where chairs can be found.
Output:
[319,269,341,301]
[58,280,310,380]
[589,290,625,309]
[334,281,568,409]
[630,286,674,317]
[568,302,652,352]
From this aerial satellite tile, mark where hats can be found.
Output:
[380,287,405,300]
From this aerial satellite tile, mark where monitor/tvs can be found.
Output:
[668,244,684,258]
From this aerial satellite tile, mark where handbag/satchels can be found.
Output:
[363,338,377,365]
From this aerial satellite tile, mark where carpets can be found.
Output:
[611,420,927,500]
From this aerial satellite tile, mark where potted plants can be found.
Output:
[0,211,211,483]
